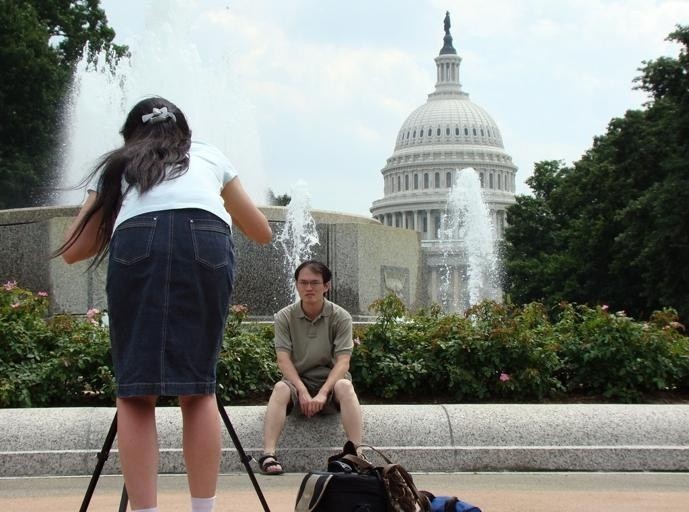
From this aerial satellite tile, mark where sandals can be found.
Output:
[259,452,284,474]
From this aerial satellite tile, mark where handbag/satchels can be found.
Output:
[294,440,432,512]
[418,490,482,512]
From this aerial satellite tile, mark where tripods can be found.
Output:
[78,385,271,511]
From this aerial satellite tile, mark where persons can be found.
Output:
[259,258,364,477]
[47,95,274,512]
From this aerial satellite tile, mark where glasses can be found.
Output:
[298,280,323,287]
